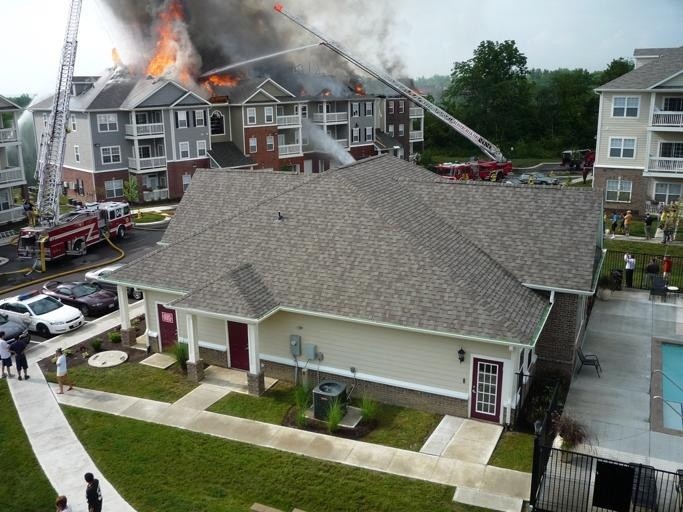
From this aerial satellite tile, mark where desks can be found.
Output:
[667,286,679,299]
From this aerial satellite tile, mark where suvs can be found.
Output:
[501,177,524,183]
[519,172,558,185]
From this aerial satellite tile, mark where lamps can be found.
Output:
[457,345,465,363]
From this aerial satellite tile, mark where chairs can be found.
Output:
[648,275,669,303]
[577,345,602,378]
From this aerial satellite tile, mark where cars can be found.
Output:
[84,264,144,301]
[0,312,31,356]
[40,279,118,318]
[0,292,85,337]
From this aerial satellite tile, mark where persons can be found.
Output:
[582,167,588,184]
[610,204,670,240]
[645,259,659,289]
[490,171,497,183]
[623,252,636,289]
[56,349,73,395]
[84,473,102,512]
[0,332,15,378]
[549,169,556,176]
[461,173,469,180]
[24,199,36,227]
[55,496,72,512]
[8,334,29,381]
[529,175,535,184]
[662,255,673,287]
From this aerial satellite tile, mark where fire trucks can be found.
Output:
[15,0,134,266]
[272,2,513,180]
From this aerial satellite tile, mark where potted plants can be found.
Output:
[550,408,599,463]
[599,274,621,301]
[174,342,193,373]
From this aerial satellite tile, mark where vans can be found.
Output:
[560,148,593,164]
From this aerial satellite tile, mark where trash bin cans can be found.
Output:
[609,269,623,292]
